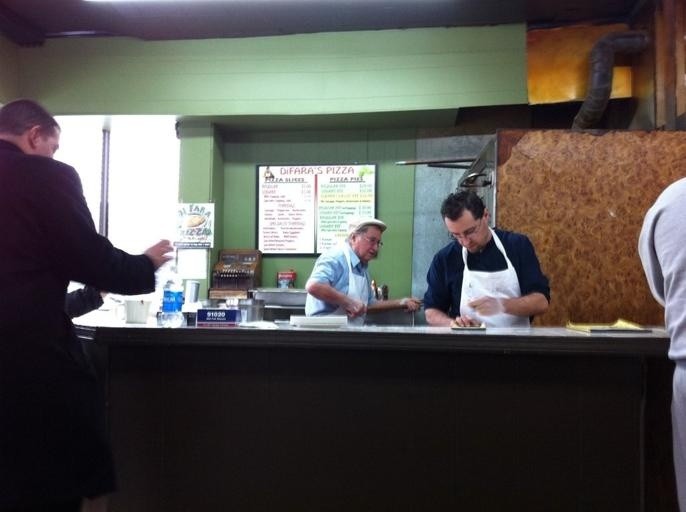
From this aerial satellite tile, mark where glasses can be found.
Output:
[447,219,482,239]
[359,232,384,247]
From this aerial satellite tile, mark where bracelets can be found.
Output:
[399,298,407,309]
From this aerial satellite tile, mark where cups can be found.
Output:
[115,301,152,324]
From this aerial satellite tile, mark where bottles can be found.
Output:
[161,265,185,328]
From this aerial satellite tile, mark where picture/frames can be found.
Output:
[255,162,378,258]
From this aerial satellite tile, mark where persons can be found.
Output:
[304,214,423,327]
[425,190,551,328]
[65,285,108,319]
[638,174,685,512]
[1,98,178,512]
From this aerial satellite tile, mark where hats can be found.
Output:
[348,217,387,240]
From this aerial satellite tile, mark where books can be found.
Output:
[289,315,348,328]
[566,318,654,335]
